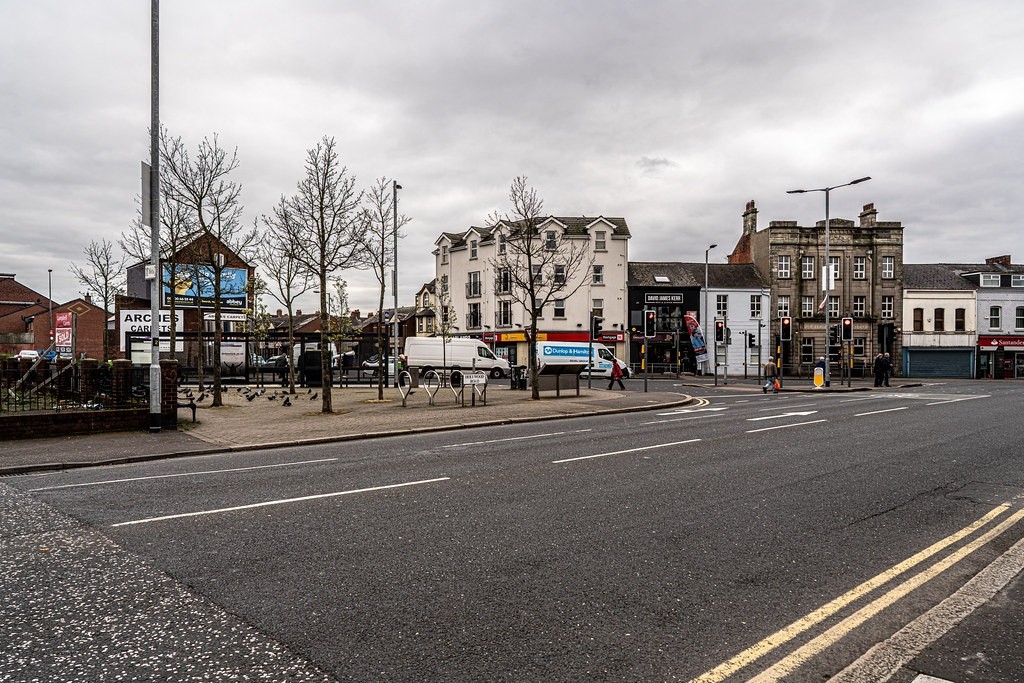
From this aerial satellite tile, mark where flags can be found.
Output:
[684,315,708,363]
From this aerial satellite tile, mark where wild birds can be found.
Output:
[308,388,311,394]
[409,391,416,396]
[268,390,289,401]
[243,386,266,401]
[205,386,228,398]
[282,396,291,406]
[178,388,193,397]
[196,393,205,402]
[237,388,241,392]
[310,393,318,400]
[295,392,298,399]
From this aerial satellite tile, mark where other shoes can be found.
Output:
[774,392,778,394]
[763,386,767,394]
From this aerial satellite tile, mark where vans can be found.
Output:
[405,337,511,379]
[292,342,337,374]
[535,341,629,379]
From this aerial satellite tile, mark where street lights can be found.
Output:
[392,183,403,386]
[313,291,331,350]
[48,269,52,350]
[704,244,717,373]
[786,176,871,386]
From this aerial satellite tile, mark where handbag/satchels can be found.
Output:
[774,378,781,390]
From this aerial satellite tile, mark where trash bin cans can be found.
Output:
[409,367,419,388]
[511,365,527,390]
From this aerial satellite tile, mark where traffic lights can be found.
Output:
[593,316,603,340]
[830,325,838,343]
[749,333,755,348]
[645,310,656,338]
[781,317,792,341]
[715,321,724,342]
[842,318,853,341]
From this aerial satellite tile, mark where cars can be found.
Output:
[15,350,57,362]
[253,354,290,367]
[621,368,634,379]
[364,356,395,378]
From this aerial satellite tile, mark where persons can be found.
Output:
[874,352,893,387]
[689,323,704,349]
[400,354,407,387]
[763,356,778,394]
[816,356,825,374]
[342,352,348,375]
[606,359,625,390]
[275,353,288,379]
